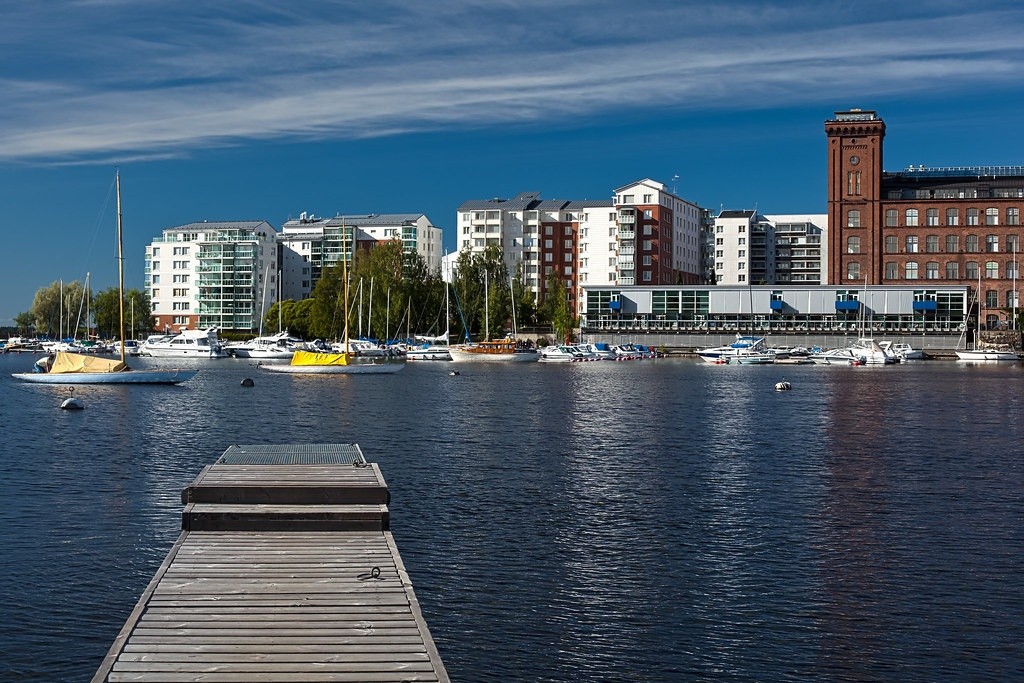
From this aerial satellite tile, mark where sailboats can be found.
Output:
[540,274,924,366]
[956,266,1019,360]
[12,165,202,386]
[449,265,539,364]
[256,215,404,375]
[2,268,445,362]
